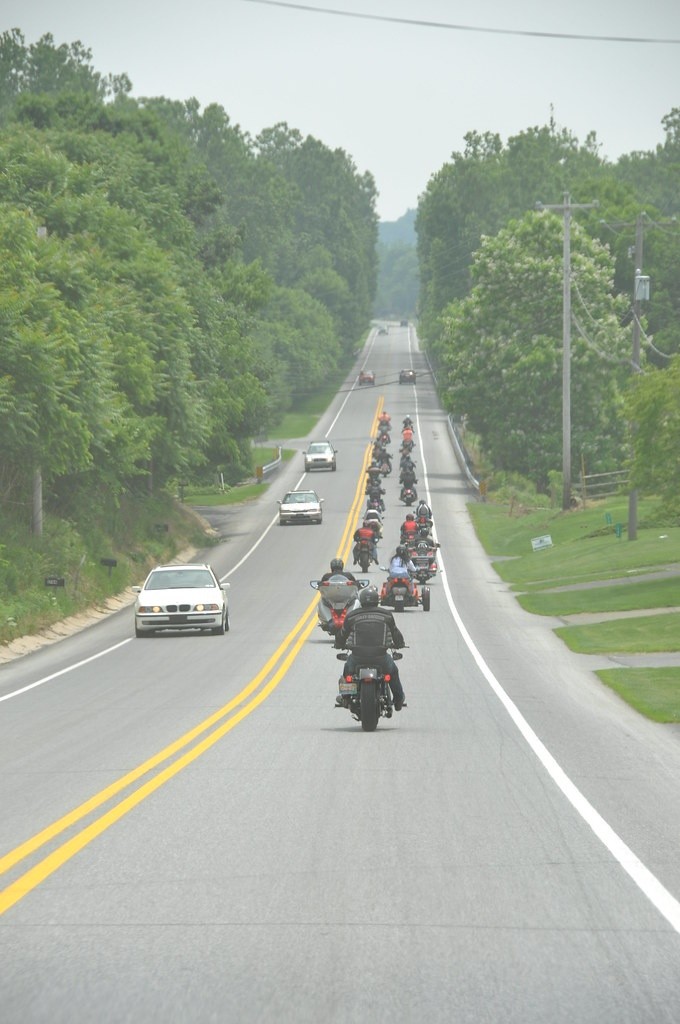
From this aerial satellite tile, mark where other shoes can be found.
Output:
[336,695,350,708]
[394,693,405,711]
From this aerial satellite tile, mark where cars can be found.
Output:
[398,369,417,384]
[131,564,230,638]
[400,320,408,327]
[358,371,375,385]
[378,325,389,335]
[276,490,324,526]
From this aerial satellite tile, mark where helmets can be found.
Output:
[368,413,413,506]
[331,559,343,570]
[396,545,406,554]
[419,500,426,505]
[369,502,375,508]
[363,520,370,528]
[360,588,378,606]
[420,528,429,536]
[406,514,414,521]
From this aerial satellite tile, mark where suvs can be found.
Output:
[303,441,338,471]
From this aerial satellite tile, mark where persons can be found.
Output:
[399,415,418,498]
[365,503,384,538]
[400,514,419,544]
[415,499,433,527]
[364,460,385,511]
[372,412,391,456]
[377,448,393,472]
[334,587,405,712]
[386,543,416,592]
[415,529,435,548]
[318,558,362,625]
[353,520,378,565]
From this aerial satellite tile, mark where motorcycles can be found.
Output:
[331,643,409,731]
[379,566,430,612]
[309,579,369,636]
[353,412,442,585]
